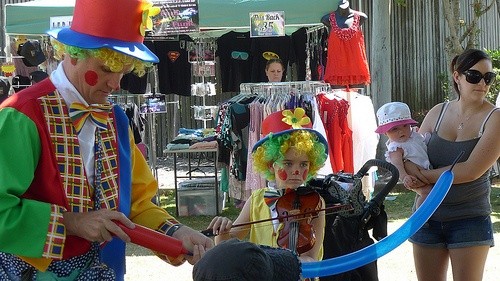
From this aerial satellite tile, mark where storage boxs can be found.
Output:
[177,178,227,216]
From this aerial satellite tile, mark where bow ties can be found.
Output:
[71,100,114,133]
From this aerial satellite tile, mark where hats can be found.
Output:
[17,40,46,68]
[0,71,50,102]
[249,108,328,165]
[194,240,303,281]
[120,72,146,94]
[45,0,163,64]
[374,102,419,133]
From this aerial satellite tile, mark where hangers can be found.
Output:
[231,82,330,103]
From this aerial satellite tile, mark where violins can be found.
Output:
[276,187,322,254]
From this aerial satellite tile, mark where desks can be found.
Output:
[163,134,221,217]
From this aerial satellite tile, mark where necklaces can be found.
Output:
[456,111,475,130]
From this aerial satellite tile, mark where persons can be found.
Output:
[208,107,328,281]
[321,0,368,88]
[404,49,500,281]
[192,238,302,281]
[375,102,432,210]
[0,0,216,281]
[265,58,285,83]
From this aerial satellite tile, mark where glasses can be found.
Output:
[463,70,497,86]
